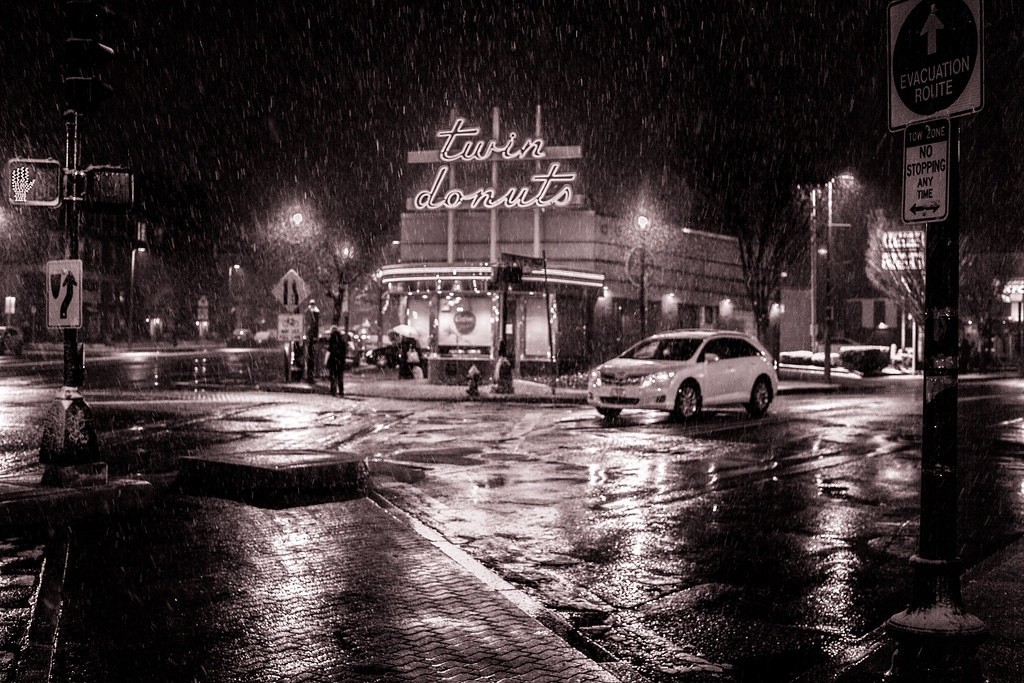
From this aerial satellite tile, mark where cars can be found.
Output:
[225,328,276,347]
[0,325,25,357]
[586,328,780,422]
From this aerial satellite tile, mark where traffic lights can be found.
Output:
[84,165,136,213]
[7,157,63,208]
[491,262,522,284]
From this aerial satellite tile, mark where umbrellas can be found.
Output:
[393,324,420,340]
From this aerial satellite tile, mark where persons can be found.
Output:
[325,324,347,397]
[397,336,415,381]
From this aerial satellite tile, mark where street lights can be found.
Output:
[822,171,855,384]
[127,241,146,351]
[226,261,240,337]
[637,215,652,341]
